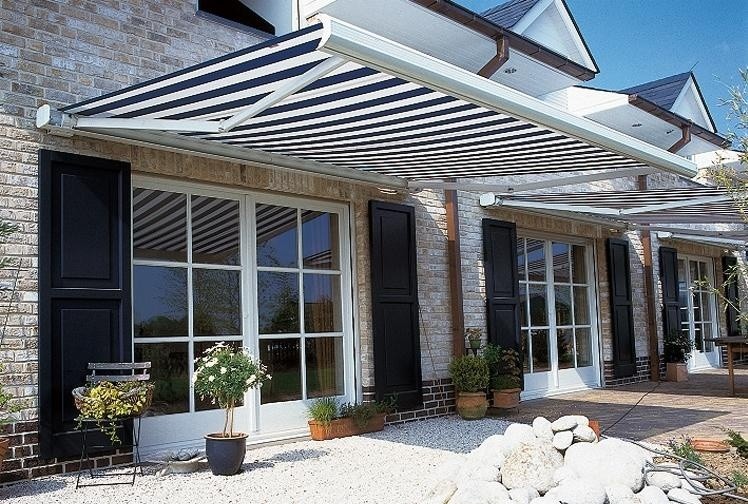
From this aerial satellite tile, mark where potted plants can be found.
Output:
[663,334,698,382]
[115,380,155,417]
[493,374,522,409]
[0,362,30,472]
[463,328,482,348]
[447,353,490,419]
[72,379,122,449]
[308,393,389,441]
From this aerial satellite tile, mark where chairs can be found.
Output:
[75,361,152,490]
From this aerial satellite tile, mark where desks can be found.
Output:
[703,336,748,397]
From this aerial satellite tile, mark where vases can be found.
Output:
[203,431,250,476]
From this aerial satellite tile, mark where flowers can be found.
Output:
[191,340,273,438]
